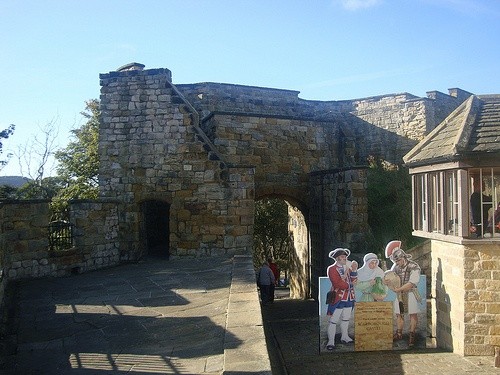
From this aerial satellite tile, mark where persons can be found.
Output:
[256,261,276,303]
[491,202,500,237]
[355,253,388,303]
[470,177,494,236]
[325,248,359,350]
[383,241,422,346]
[267,258,280,282]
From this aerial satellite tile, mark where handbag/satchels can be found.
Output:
[257,278,261,287]
